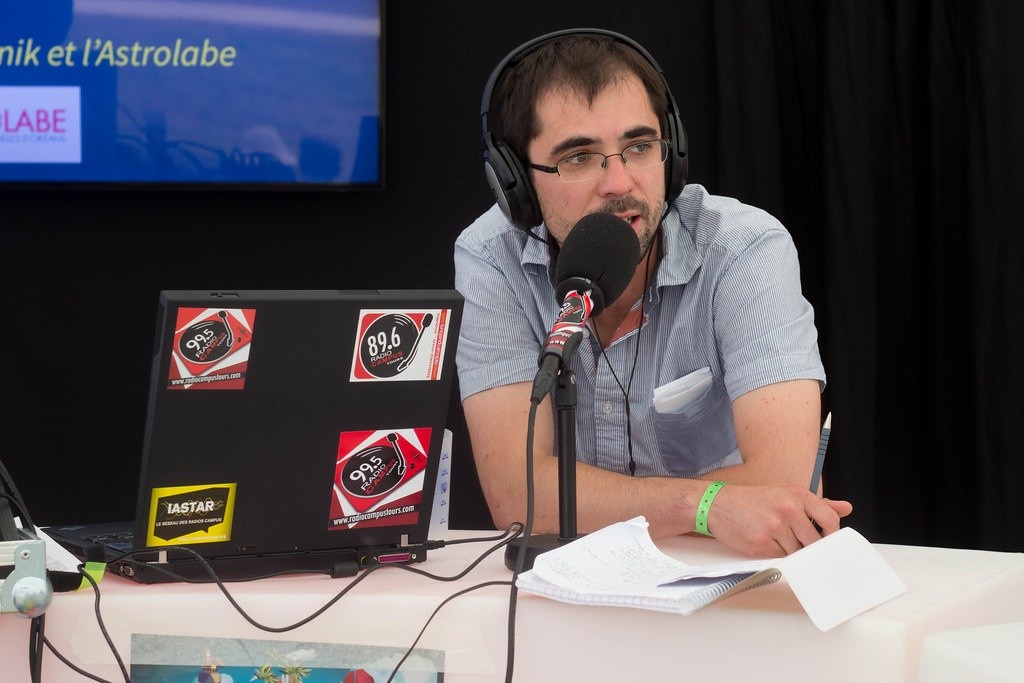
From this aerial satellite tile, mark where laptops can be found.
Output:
[33,290,464,585]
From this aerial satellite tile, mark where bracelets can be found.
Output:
[694,477,727,540]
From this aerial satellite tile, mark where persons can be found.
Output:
[450,29,853,560]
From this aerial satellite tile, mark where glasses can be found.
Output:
[525,132,672,183]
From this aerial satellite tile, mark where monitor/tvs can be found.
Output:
[0,0,389,196]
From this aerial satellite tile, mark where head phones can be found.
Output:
[480,27,689,231]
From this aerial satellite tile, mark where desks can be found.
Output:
[1,529,1024,683]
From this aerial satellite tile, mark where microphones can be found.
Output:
[529,211,641,404]
